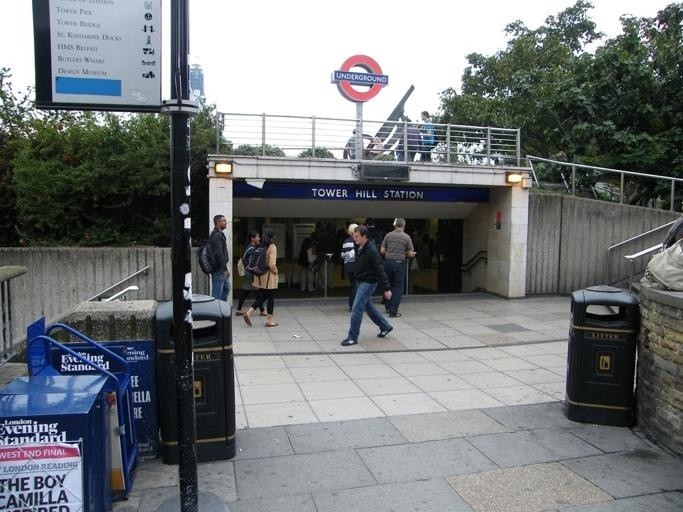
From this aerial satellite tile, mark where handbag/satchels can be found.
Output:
[645,239,683,293]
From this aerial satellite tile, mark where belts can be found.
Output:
[382,257,406,264]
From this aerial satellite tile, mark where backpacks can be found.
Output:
[247,244,272,278]
[297,238,316,269]
[197,230,228,275]
[237,257,247,279]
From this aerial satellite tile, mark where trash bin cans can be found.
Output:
[565,284,641,427]
[154,293,236,465]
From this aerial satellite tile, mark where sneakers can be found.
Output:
[386,306,400,317]
[377,324,394,338]
[341,338,358,346]
[300,288,316,292]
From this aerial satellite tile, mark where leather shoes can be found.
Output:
[242,312,251,325]
[260,311,268,317]
[236,311,244,316]
[265,322,279,327]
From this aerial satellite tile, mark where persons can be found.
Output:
[235,230,267,316]
[242,230,279,327]
[398,119,422,161]
[348,129,364,159]
[340,226,392,346]
[420,111,434,162]
[206,216,230,300]
[341,223,359,312]
[381,216,416,318]
[298,219,440,293]
[370,136,384,154]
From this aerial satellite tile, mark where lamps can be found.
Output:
[214,160,233,174]
[504,171,523,184]
